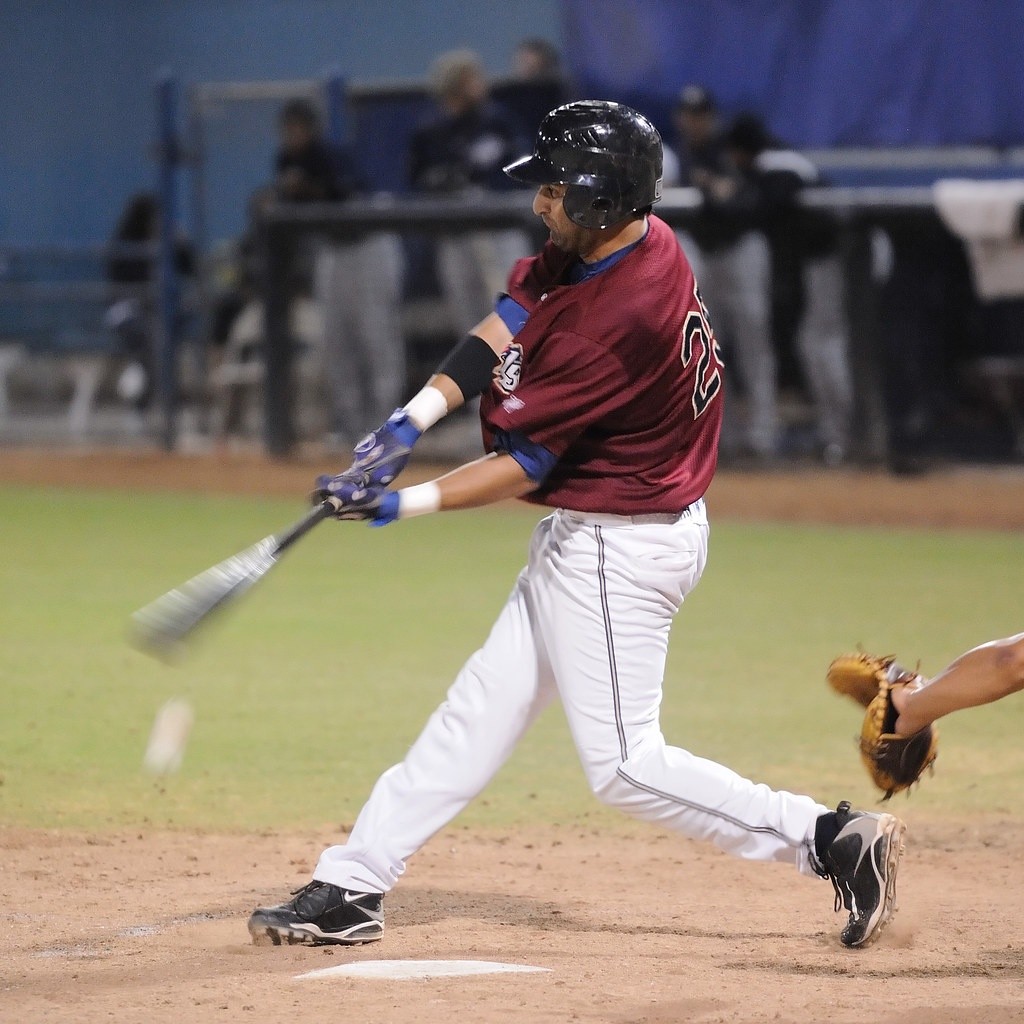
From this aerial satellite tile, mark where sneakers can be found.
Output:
[249,874,384,950]
[812,801,904,945]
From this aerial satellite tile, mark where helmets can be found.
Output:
[500,100,664,231]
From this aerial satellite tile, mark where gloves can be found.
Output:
[353,406,427,491]
[311,476,405,528]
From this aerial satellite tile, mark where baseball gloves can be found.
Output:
[827,649,938,803]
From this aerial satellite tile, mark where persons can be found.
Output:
[249,100,899,950]
[890,632,1023,739]
[71,40,853,471]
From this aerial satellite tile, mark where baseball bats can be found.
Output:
[129,492,337,659]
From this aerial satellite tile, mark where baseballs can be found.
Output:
[145,733,180,770]
